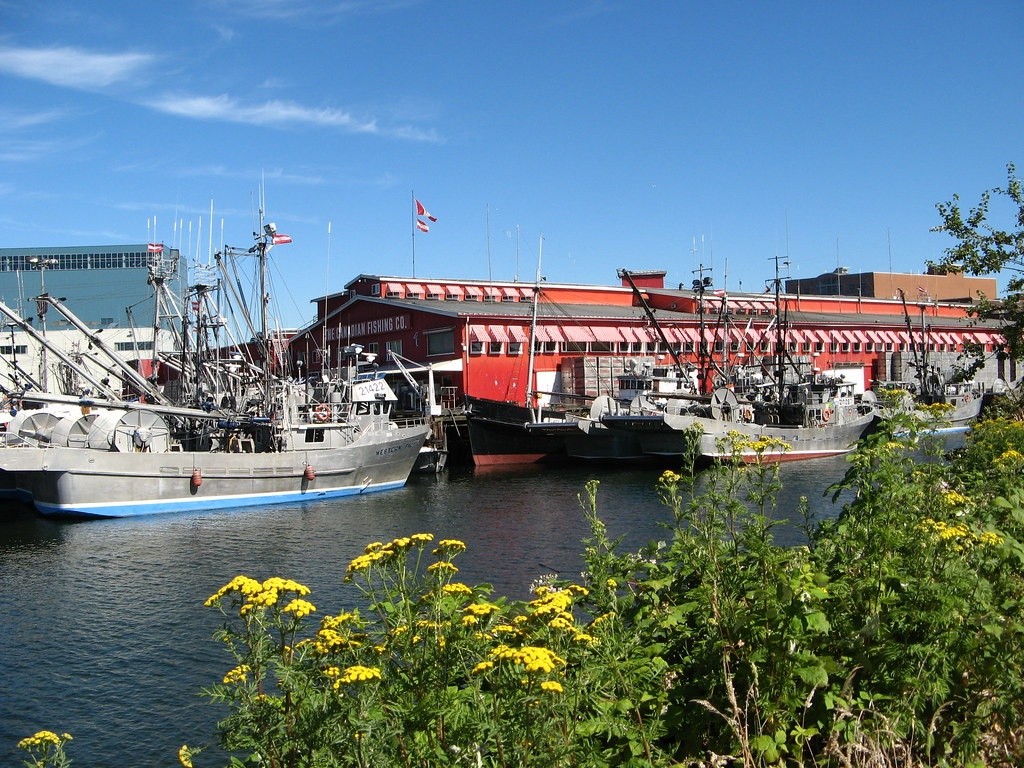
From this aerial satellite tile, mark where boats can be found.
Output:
[409,440,449,472]
[456,353,710,466]
[523,232,880,464]
[863,285,987,431]
[0,167,433,521]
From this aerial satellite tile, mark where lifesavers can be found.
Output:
[963,395,972,405]
[822,409,830,422]
[316,405,330,421]
[2,394,24,429]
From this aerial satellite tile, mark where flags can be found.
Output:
[192,301,198,310]
[149,244,163,252]
[416,200,437,222]
[714,290,724,296]
[417,219,429,232]
[272,234,292,244]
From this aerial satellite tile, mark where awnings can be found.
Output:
[520,289,534,297]
[465,287,483,296]
[502,288,520,297]
[472,325,651,342]
[406,284,425,294]
[698,301,777,310]
[647,328,831,343]
[830,330,962,344]
[962,333,1024,345]
[386,283,405,293]
[483,287,502,296]
[446,286,464,295]
[426,284,445,294]
